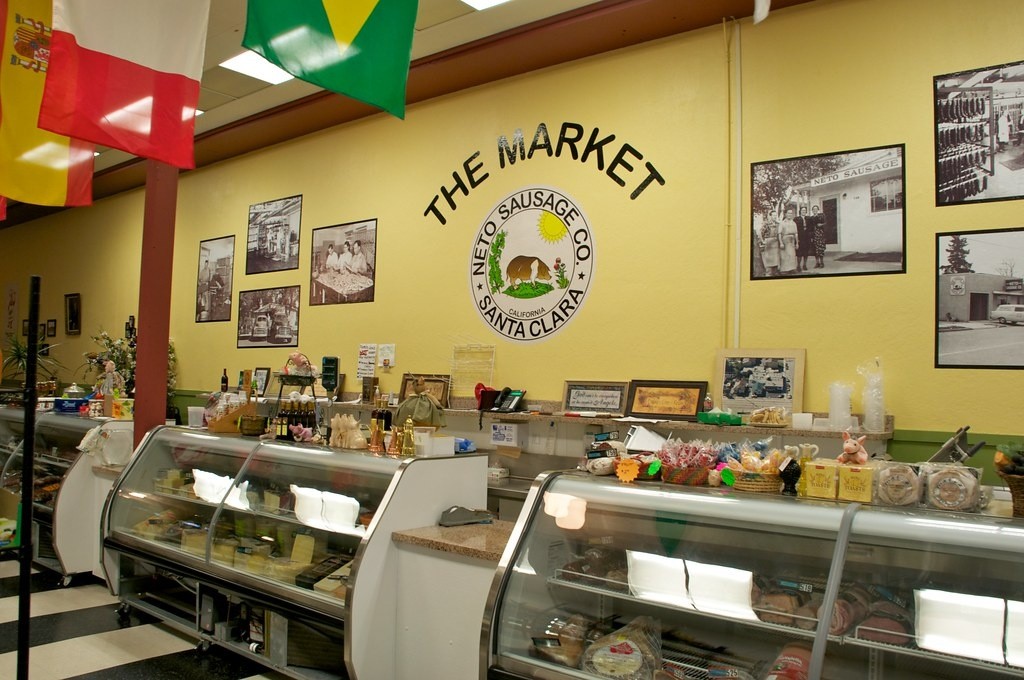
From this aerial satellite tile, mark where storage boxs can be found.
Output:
[188,406,205,428]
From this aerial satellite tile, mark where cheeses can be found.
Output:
[132,469,315,583]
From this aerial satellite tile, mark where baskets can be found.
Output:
[239,389,267,436]
[613,457,658,480]
[727,467,783,492]
[660,464,711,486]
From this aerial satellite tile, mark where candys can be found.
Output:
[660,439,790,474]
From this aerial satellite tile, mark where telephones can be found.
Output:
[486,387,526,413]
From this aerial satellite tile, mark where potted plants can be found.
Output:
[2,331,69,388]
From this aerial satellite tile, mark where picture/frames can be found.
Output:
[195,235,234,322]
[309,217,378,306]
[252,366,271,396]
[750,143,906,282]
[245,193,303,276]
[63,292,83,334]
[624,379,708,423]
[236,285,301,347]
[400,373,449,410]
[719,348,805,420]
[934,228,1024,371]
[933,59,1024,208]
[23,318,56,356]
[562,378,630,417]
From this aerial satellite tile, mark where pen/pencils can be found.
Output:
[530,412,552,415]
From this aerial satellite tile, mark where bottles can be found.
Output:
[221,369,228,392]
[370,399,393,434]
[274,401,317,442]
[250,376,258,397]
[237,370,244,390]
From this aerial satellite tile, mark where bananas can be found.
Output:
[750,409,783,423]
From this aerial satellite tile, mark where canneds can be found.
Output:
[20,380,57,397]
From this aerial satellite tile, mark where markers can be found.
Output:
[565,412,597,418]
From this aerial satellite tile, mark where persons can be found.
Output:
[758,205,827,276]
[198,259,222,313]
[995,106,1024,151]
[325,239,369,276]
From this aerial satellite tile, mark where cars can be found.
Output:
[251,315,270,340]
[269,313,293,343]
[990,303,1024,324]
[238,325,252,339]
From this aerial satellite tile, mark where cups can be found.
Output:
[413,426,436,459]
[117,398,135,421]
